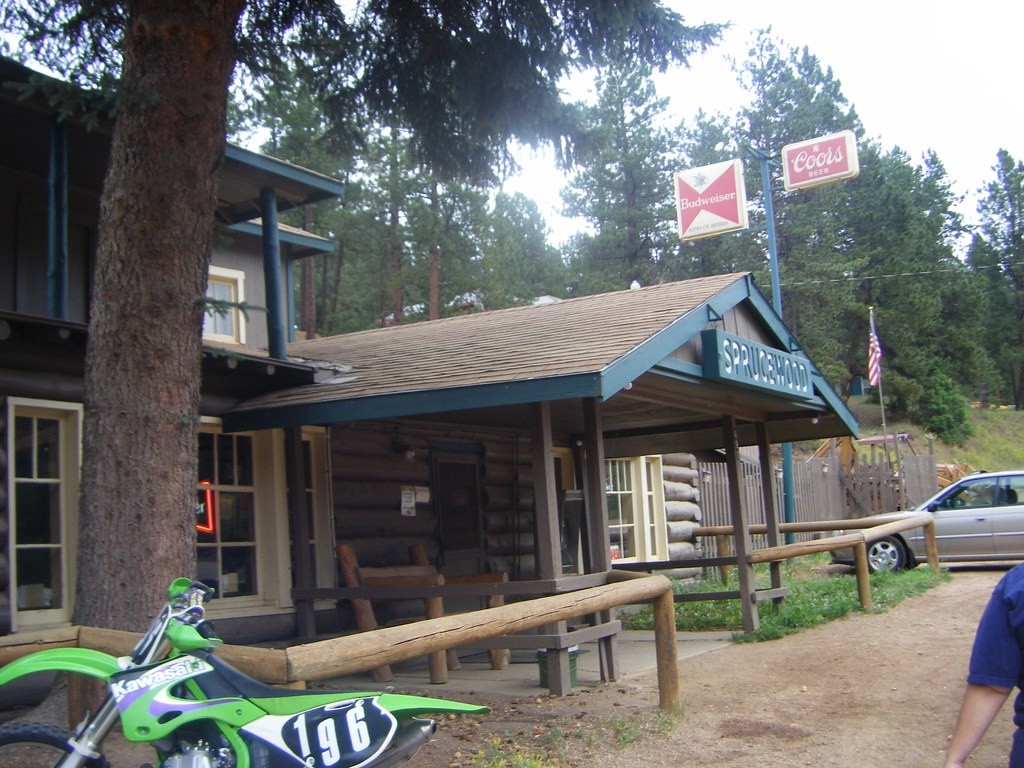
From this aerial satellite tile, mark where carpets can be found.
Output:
[460,650,590,663]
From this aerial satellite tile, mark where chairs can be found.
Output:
[337,542,509,684]
[998,489,1017,505]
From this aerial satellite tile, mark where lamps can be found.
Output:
[391,441,416,460]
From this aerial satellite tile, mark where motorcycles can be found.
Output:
[0,574,496,768]
[829,468,1024,575]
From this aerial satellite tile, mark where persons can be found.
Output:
[971,470,991,505]
[945,562,1024,768]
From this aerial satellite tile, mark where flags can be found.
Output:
[869,323,881,387]
